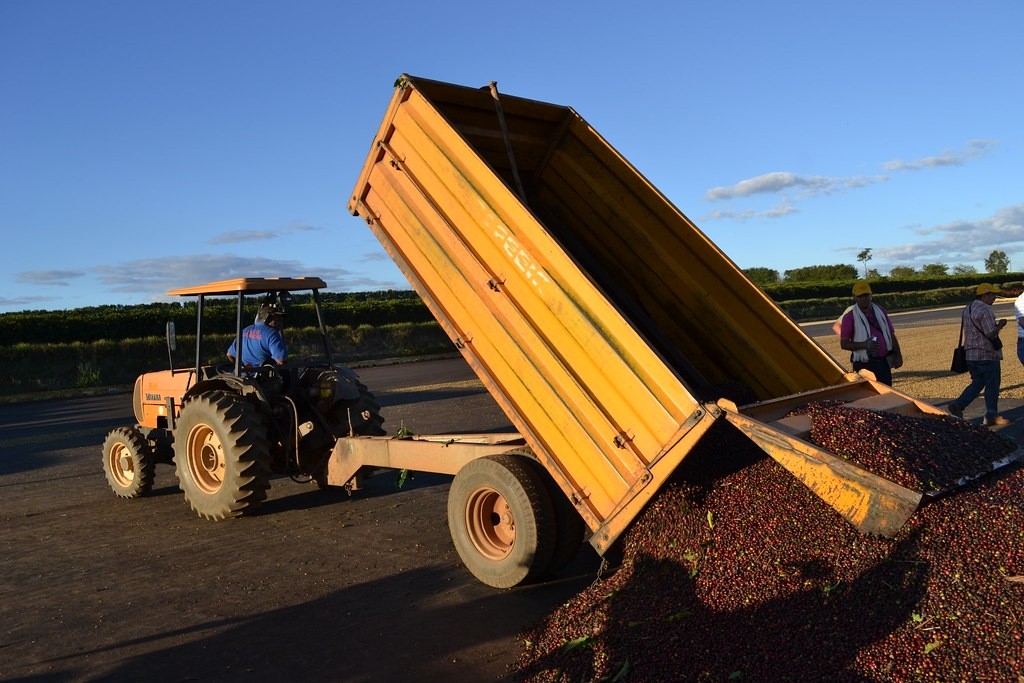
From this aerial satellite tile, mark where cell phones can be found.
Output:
[872,336,878,341]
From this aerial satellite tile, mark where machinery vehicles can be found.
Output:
[103,74,1023,592]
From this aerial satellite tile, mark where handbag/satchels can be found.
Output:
[991,336,1002,350]
[950,346,969,373]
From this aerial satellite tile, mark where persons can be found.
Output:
[840,281,903,387]
[227,307,288,377]
[1014,292,1024,366]
[949,283,1010,426]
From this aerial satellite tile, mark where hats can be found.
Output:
[853,282,872,296]
[976,283,1001,295]
[260,306,287,323]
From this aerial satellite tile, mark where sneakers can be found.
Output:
[983,415,1010,425]
[948,404,965,420]
